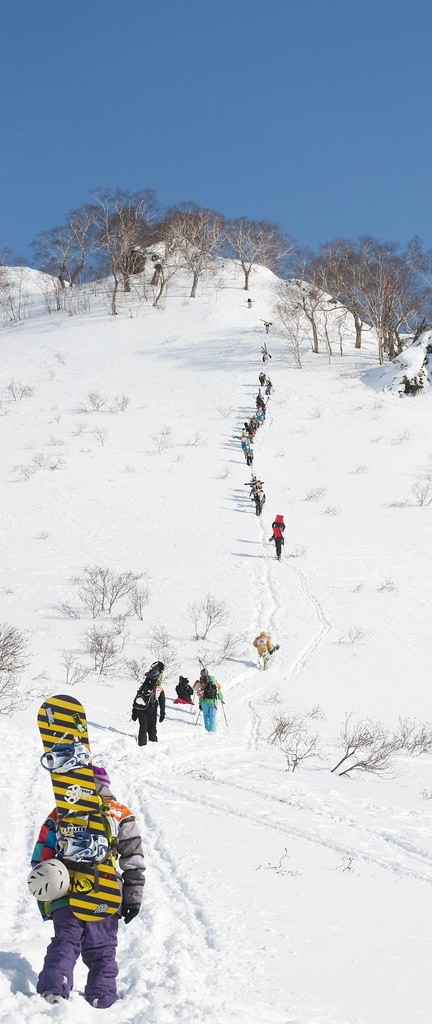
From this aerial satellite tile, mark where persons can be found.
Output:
[272,519,286,532]
[29,763,146,1009]
[175,674,194,704]
[199,669,224,732]
[135,677,166,746]
[269,528,285,562]
[253,631,273,671]
[233,294,275,515]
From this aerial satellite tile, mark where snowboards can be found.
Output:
[36,694,122,923]
[272,515,284,545]
[132,661,165,711]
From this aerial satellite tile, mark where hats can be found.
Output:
[157,679,161,686]
[200,668,209,676]
[92,765,110,787]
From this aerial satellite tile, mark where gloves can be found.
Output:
[159,715,165,722]
[120,907,139,924]
[132,713,138,721]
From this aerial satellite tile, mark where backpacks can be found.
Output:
[203,681,218,699]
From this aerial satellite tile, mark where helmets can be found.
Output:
[27,858,70,902]
